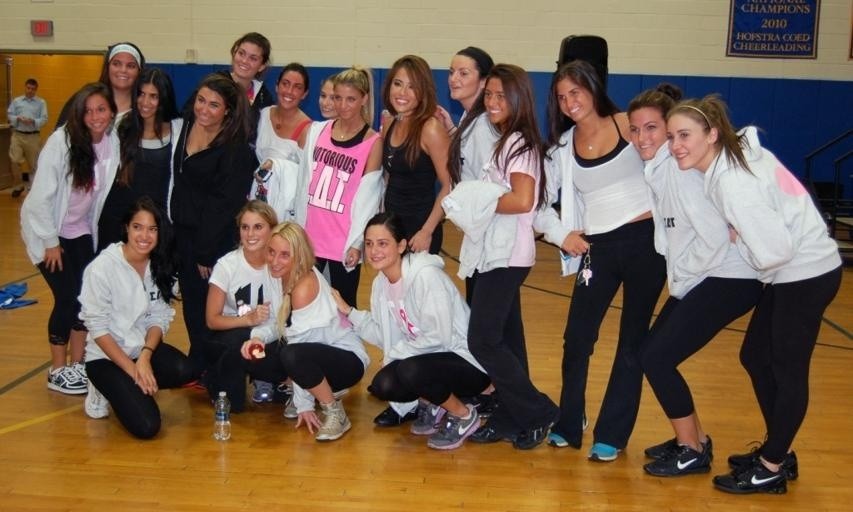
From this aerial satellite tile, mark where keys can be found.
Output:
[576,255,592,286]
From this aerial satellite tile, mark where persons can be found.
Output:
[441,64,561,450]
[295,65,383,308]
[379,54,452,255]
[241,221,370,442]
[217,32,274,141]
[20,82,120,395]
[206,201,294,416]
[666,93,843,495]
[435,45,500,182]
[318,72,339,119]
[628,83,765,476]
[173,75,259,393]
[531,61,666,460]
[76,192,197,438]
[331,212,491,450]
[8,78,48,197]
[54,41,146,128]
[115,68,183,227]
[249,63,314,224]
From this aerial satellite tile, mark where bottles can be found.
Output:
[288,150,299,163]
[483,162,511,191]
[214,391,231,440]
[238,300,252,316]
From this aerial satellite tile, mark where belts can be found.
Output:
[15,129,41,135]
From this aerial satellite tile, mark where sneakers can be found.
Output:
[426,403,485,452]
[468,417,518,444]
[412,402,449,436]
[712,459,788,497]
[513,411,557,449]
[643,444,712,479]
[588,443,623,462]
[281,384,299,420]
[548,416,589,448]
[83,376,110,421]
[11,186,26,198]
[373,406,420,428]
[477,390,501,414]
[249,379,274,405]
[46,366,90,395]
[727,434,800,482]
[645,434,713,462]
[72,362,91,385]
[315,398,352,443]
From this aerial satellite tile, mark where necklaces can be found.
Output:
[339,118,365,139]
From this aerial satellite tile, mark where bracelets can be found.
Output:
[141,346,154,353]
[447,125,456,133]
